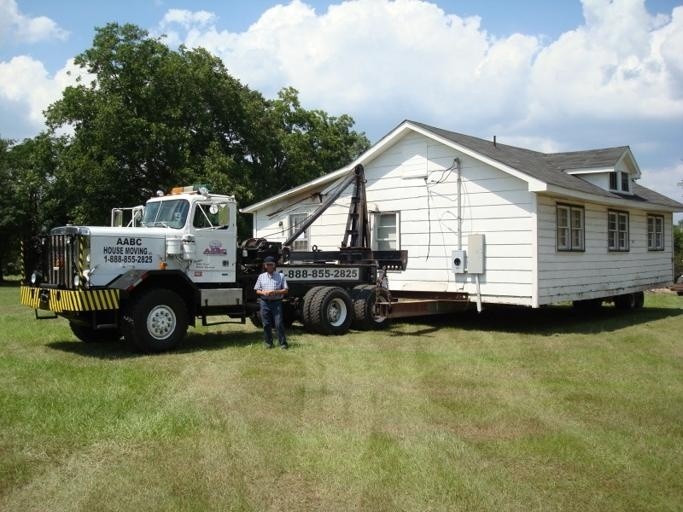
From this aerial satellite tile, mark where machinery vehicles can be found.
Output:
[15,164,413,355]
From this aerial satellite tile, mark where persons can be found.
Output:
[253,256,289,349]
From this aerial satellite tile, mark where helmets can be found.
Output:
[263,257,276,271]
